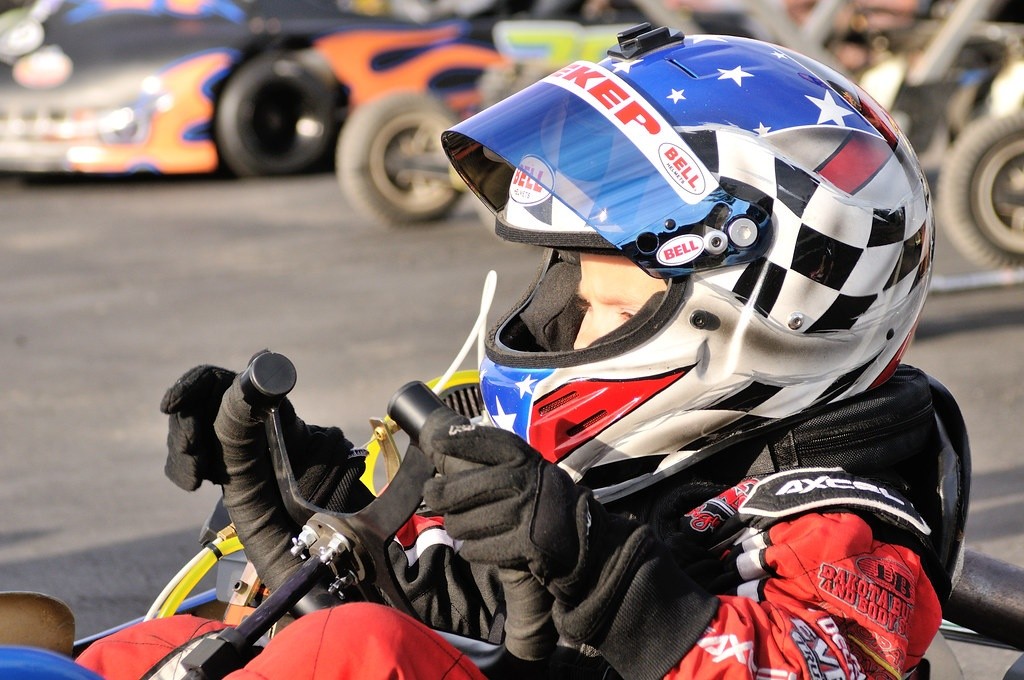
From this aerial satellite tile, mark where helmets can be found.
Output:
[440,22,935,506]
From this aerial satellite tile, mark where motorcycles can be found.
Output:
[316,13,705,225]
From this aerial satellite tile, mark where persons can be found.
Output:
[72,33,972,680]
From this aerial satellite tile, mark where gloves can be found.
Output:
[422,425,720,679]
[160,364,354,492]
